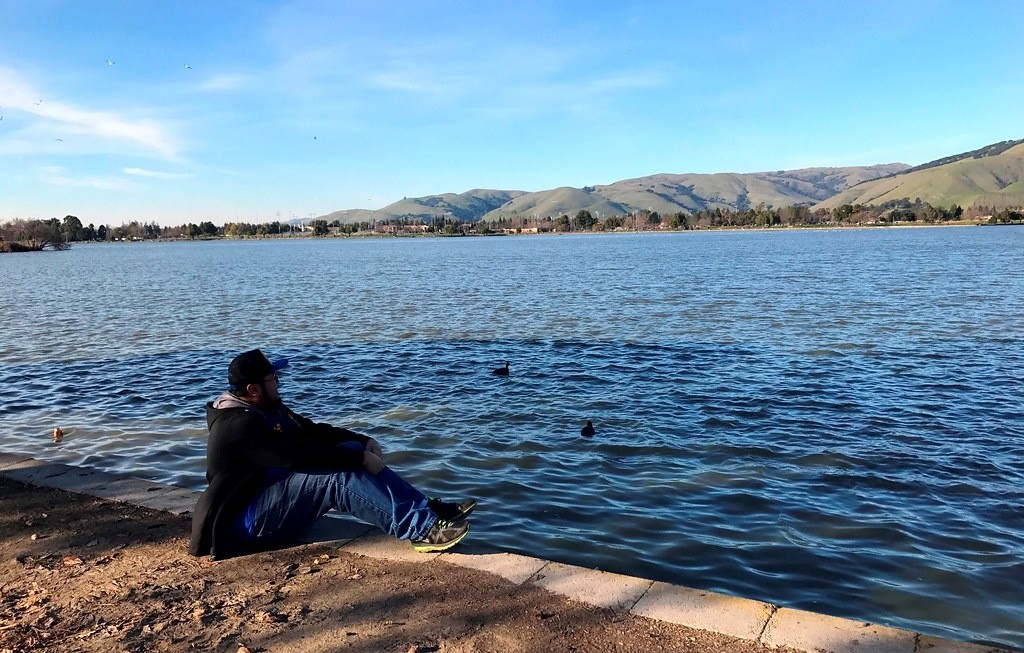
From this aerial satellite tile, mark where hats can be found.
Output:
[227,349,289,391]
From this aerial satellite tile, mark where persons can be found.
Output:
[188,349,476,562]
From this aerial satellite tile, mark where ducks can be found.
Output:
[582,420,595,437]
[491,360,513,376]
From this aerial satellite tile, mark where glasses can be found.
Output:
[260,372,278,383]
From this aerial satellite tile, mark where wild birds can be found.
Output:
[55,138,64,142]
[182,62,192,71]
[106,58,115,67]
[0,116,3,123]
[32,95,43,106]
[313,136,317,139]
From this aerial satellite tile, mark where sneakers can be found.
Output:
[409,497,477,553]
